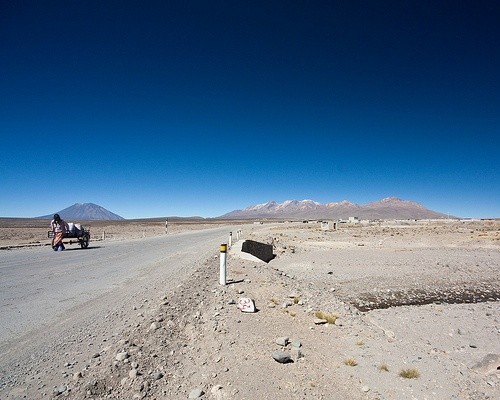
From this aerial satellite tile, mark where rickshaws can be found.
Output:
[47,220,91,250]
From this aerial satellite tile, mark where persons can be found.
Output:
[50,214,69,251]
